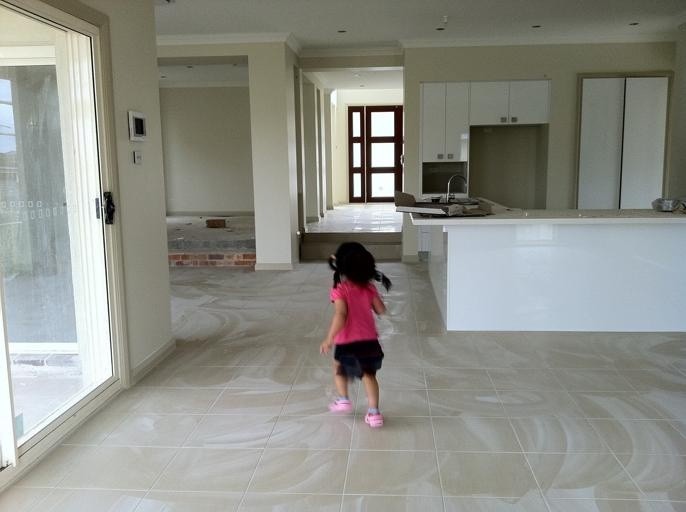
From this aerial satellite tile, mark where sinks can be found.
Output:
[425,197,495,208]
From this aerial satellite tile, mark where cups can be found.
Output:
[431,196,441,204]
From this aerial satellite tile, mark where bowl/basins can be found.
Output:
[655,197,679,212]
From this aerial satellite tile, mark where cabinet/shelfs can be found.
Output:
[417,80,550,199]
[575,71,673,210]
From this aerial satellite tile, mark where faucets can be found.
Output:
[445,174,468,202]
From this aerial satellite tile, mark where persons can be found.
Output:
[319,240,394,428]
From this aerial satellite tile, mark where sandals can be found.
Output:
[362,412,385,429]
[327,398,353,415]
